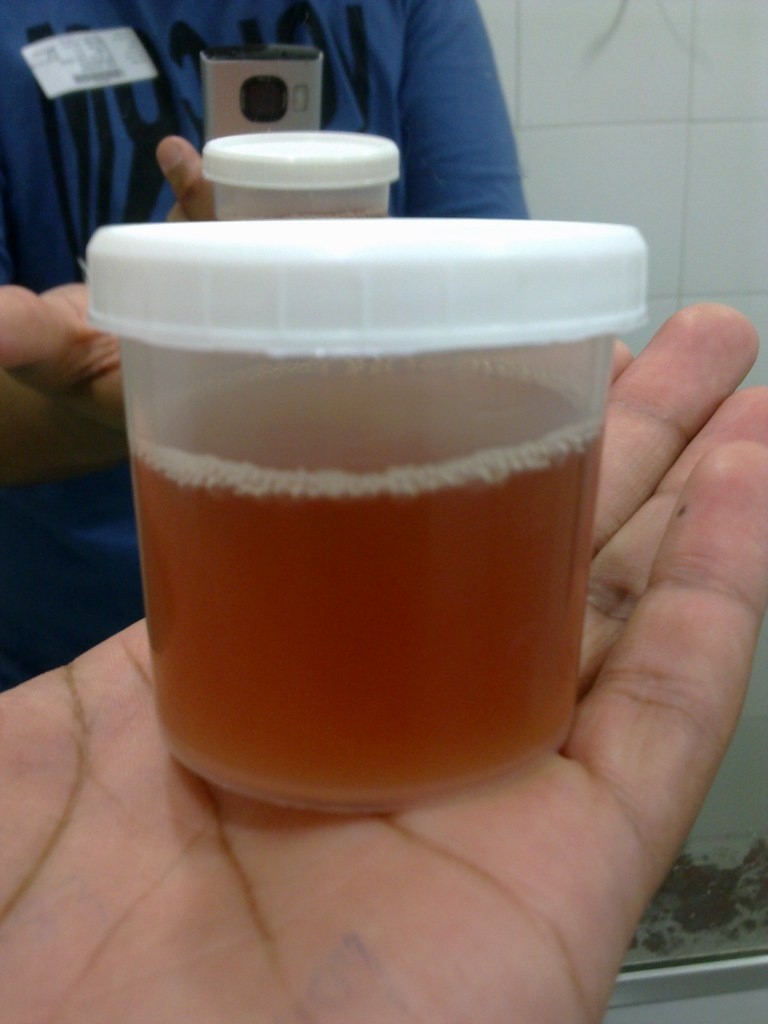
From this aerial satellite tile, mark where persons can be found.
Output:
[0,303,768,1023]
[0,0,529,691]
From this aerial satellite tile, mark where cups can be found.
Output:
[89,217,649,814]
[201,130,400,217]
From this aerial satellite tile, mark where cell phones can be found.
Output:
[200,41,324,152]
